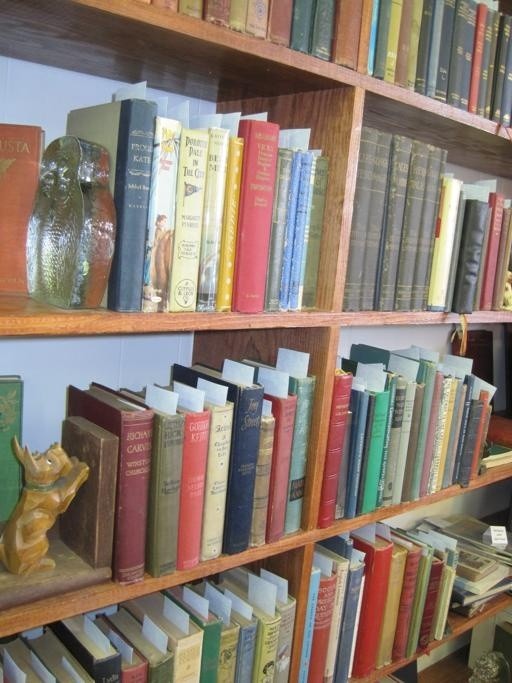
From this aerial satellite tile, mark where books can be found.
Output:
[368,0,511,123]
[153,0,364,69]
[1,568,296,682]
[61,348,314,580]
[63,100,327,314]
[0,374,24,532]
[344,126,512,312]
[301,535,459,682]
[0,125,45,306]
[322,342,496,526]
[406,513,511,620]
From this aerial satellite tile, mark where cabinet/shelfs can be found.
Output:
[0,0,511,681]
